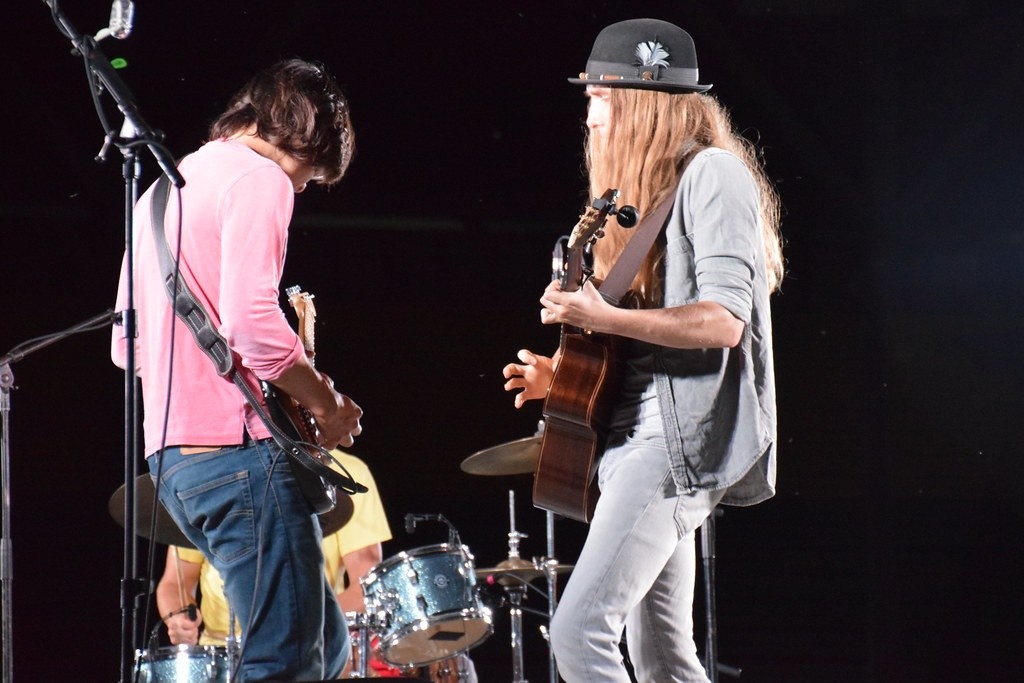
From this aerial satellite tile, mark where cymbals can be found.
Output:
[473,557,576,584]
[110,456,354,550]
[459,432,543,476]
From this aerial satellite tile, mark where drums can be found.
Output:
[359,544,495,669]
[134,645,242,683]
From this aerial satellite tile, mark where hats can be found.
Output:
[567,18,713,95]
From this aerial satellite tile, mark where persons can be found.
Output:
[154,446,389,644]
[503,18,783,683]
[109,63,368,682]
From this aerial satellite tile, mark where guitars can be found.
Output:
[532,184,647,525]
[266,280,342,514]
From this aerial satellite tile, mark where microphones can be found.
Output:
[143,603,198,663]
[473,573,505,591]
[109,1,135,40]
